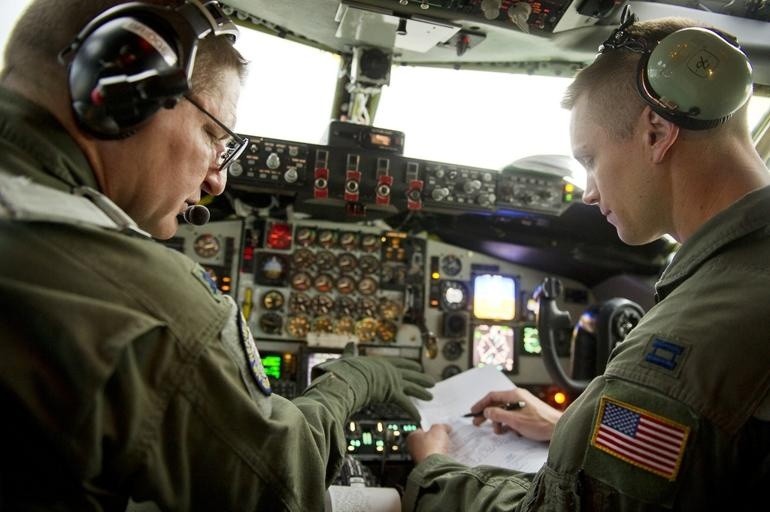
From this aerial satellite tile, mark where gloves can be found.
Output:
[314,339,434,422]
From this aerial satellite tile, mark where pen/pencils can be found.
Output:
[461,401,525,418]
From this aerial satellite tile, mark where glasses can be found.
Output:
[185,94,250,170]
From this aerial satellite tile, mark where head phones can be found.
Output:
[56,2,241,142]
[596,4,758,128]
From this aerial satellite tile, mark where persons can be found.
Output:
[0,0,436,511]
[398,18,770,512]
